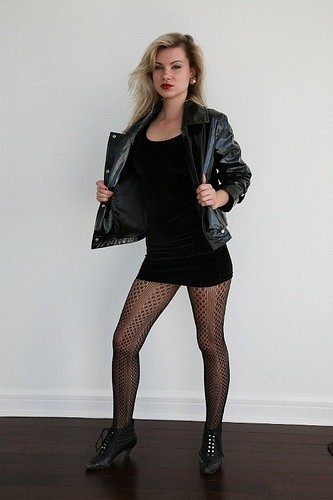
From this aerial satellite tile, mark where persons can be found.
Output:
[81,30,253,480]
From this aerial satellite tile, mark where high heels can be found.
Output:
[199,421,224,473]
[86,418,137,470]
